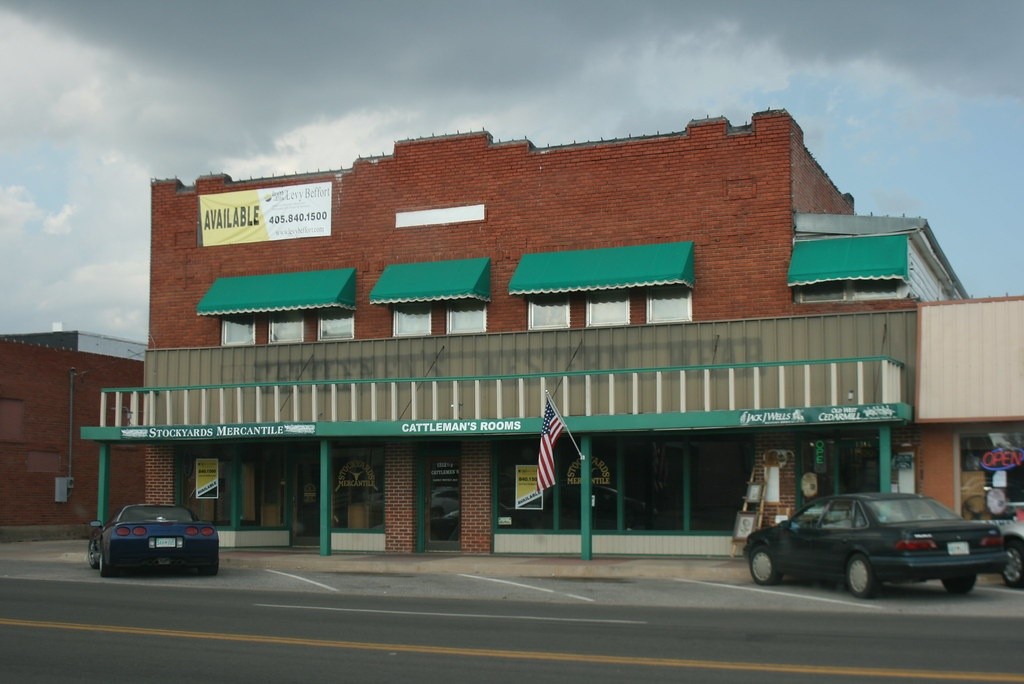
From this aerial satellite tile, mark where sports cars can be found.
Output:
[87,503,219,578]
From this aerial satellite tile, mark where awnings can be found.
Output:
[369,257,492,304]
[509,241,695,295]
[197,268,357,316]
[787,234,910,287]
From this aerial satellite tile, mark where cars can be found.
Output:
[744,493,1008,600]
[427,487,460,521]
[969,500,1024,589]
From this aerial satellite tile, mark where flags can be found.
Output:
[536,390,567,492]
